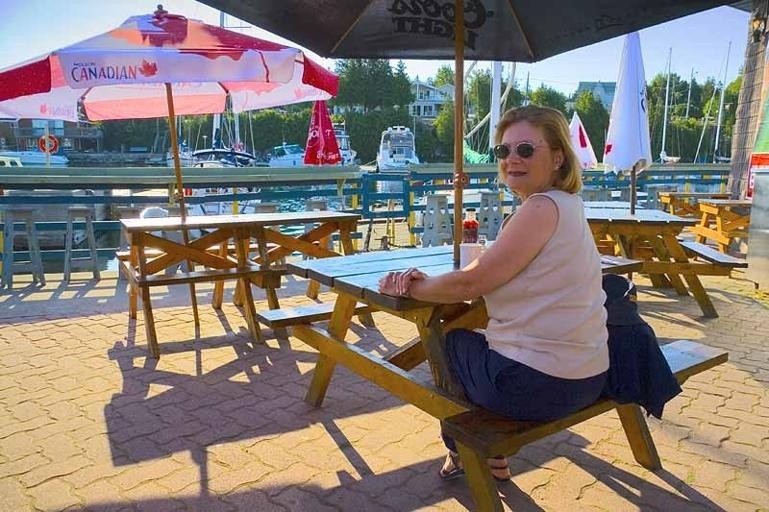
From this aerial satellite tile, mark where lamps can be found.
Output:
[753,9,769,44]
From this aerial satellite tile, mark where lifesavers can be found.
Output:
[39,136,57,154]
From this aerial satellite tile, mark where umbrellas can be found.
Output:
[567,109,597,171]
[603,31,653,281]
[0,4,340,327]
[303,99,339,166]
[195,0,742,270]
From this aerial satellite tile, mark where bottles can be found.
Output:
[461,207,480,244]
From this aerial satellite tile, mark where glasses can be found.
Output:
[492,142,545,159]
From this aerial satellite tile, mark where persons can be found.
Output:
[377,104,611,483]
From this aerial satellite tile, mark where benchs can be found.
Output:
[442,339,728,460]
[255,301,376,328]
[116,243,277,258]
[135,263,294,287]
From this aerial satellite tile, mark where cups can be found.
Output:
[460,241,483,269]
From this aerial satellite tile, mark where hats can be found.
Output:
[602,273,649,326]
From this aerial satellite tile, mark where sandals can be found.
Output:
[439,450,511,485]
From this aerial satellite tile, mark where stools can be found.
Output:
[0,208,102,291]
